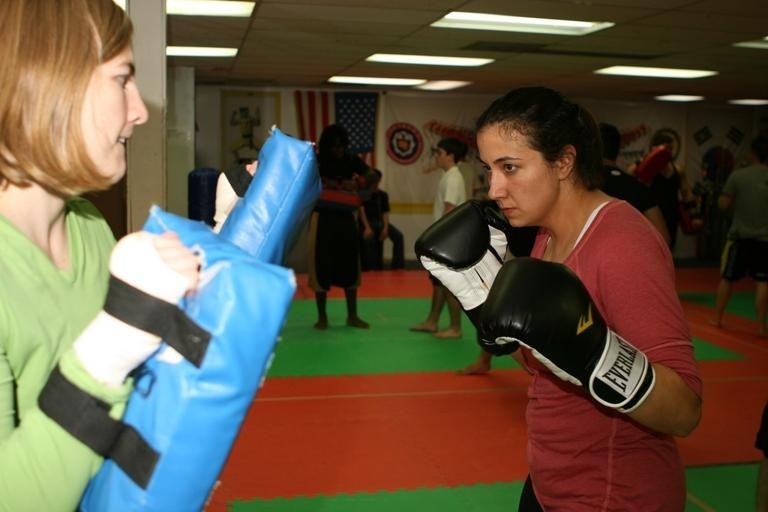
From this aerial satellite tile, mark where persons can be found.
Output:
[312,124,379,330]
[752,403,768,512]
[415,84,703,510]
[598,123,672,246]
[2,1,203,512]
[408,138,486,338]
[626,136,768,339]
[228,105,264,164]
[461,171,541,373]
[358,169,411,272]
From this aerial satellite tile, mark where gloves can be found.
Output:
[415,199,517,356]
[476,257,656,413]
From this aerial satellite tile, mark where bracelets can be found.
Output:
[384,223,388,228]
